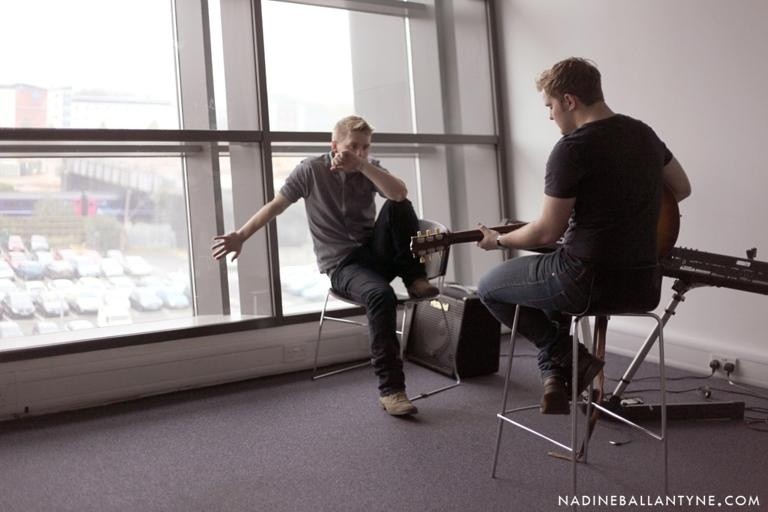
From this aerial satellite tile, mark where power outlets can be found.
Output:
[706,354,738,375]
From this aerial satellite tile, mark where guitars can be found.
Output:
[410,221,527,263]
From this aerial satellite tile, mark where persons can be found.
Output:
[211,116,440,416]
[475,56,692,414]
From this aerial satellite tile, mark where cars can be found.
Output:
[0,234,189,321]
[280,257,336,301]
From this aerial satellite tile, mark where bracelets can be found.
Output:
[497,235,507,250]
[356,159,365,172]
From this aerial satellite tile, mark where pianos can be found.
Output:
[662,248,768,295]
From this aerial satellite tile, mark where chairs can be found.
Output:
[310,218,461,402]
[489,180,680,511]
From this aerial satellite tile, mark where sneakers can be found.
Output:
[378,390,418,416]
[406,275,440,299]
[539,334,606,416]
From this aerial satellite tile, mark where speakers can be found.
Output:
[405,282,501,379]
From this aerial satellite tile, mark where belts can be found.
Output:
[326,265,336,277]
